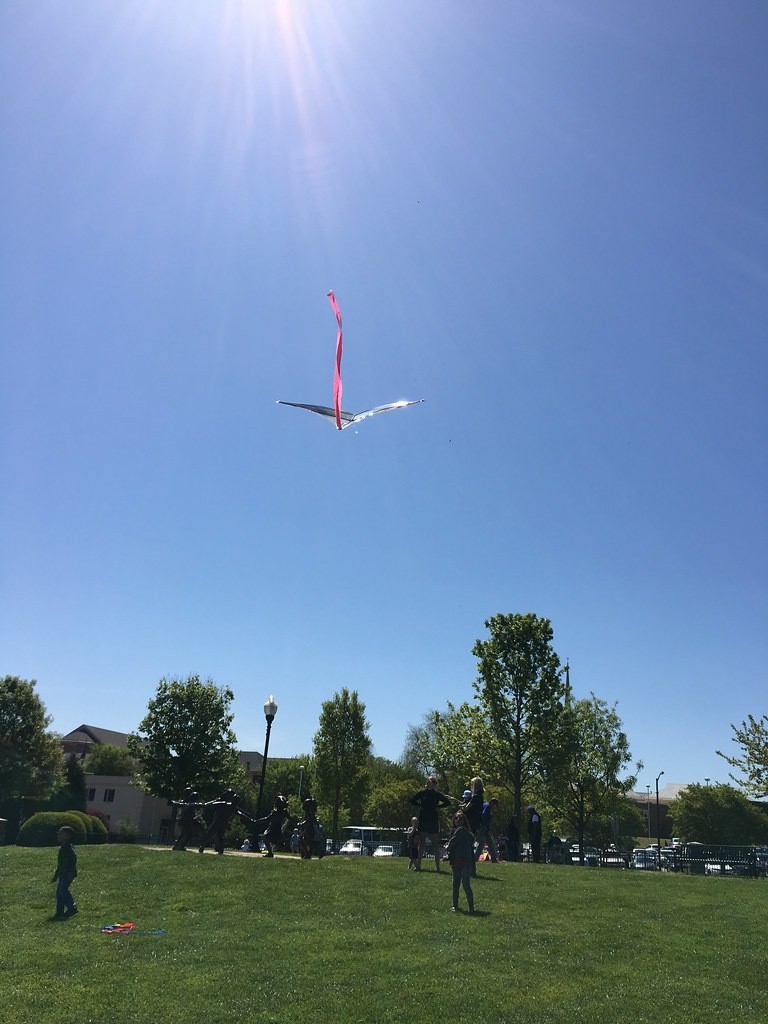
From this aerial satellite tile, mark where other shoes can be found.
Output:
[452,906,457,912]
[65,908,78,916]
[51,914,67,921]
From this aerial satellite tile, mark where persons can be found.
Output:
[169,788,327,860]
[50,826,78,921]
[241,839,251,852]
[441,810,477,912]
[289,828,298,853]
[406,776,542,873]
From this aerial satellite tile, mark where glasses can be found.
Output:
[454,817,463,820]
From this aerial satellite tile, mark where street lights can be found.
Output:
[655,772,664,870]
[705,776,711,786]
[249,696,278,852]
[298,766,307,797]
[646,784,650,837]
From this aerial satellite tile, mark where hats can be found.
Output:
[462,790,472,797]
[527,805,535,809]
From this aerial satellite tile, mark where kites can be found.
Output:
[277,292,427,429]
[101,923,168,935]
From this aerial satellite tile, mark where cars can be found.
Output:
[372,846,398,857]
[339,839,370,856]
[569,837,768,877]
